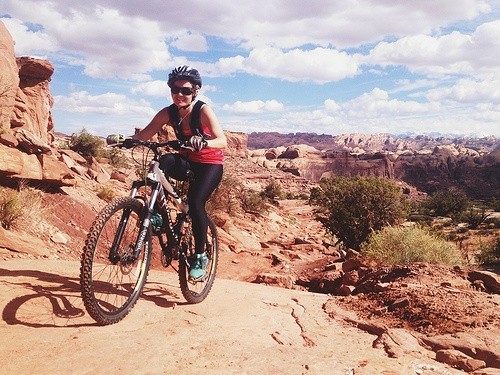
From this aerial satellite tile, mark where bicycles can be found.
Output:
[78,139,220,324]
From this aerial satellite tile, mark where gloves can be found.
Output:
[107,134,122,149]
[185,136,208,152]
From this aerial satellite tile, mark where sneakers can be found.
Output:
[151,215,162,226]
[189,251,209,281]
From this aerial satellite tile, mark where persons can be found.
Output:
[107,65,227,281]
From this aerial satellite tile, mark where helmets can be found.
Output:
[168,65,202,88]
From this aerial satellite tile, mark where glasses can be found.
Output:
[171,86,196,95]
